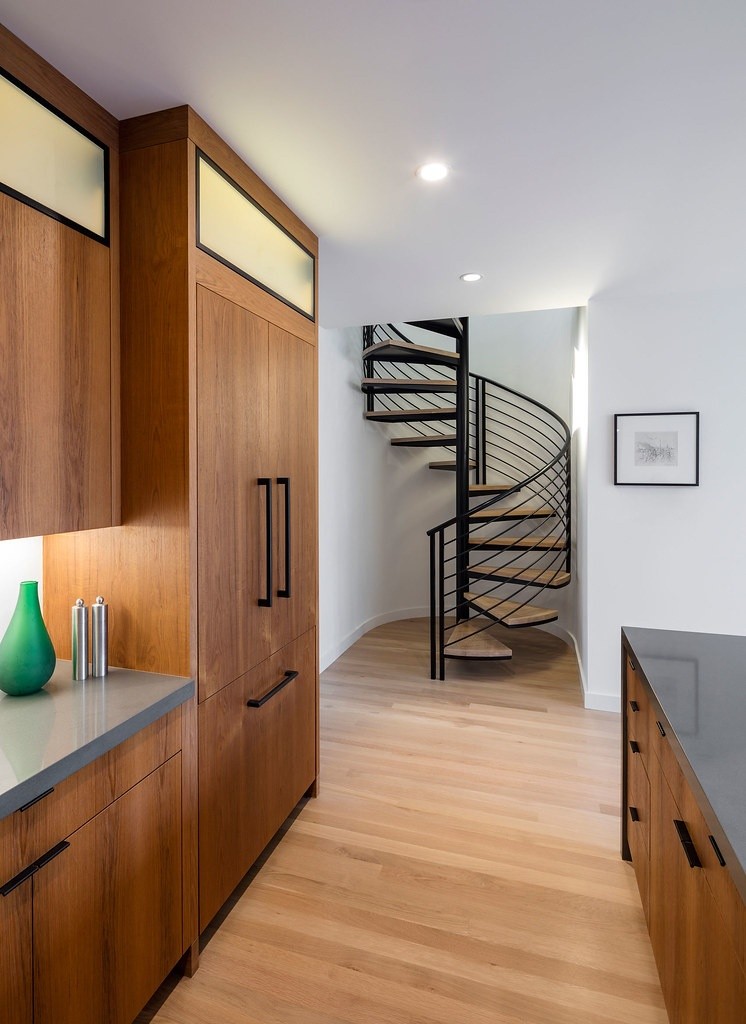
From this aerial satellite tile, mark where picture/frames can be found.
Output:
[614,412,700,486]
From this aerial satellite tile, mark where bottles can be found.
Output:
[0,581,57,695]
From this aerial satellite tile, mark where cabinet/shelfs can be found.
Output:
[0,705,191,1024]
[620,627,746,1024]
[0,23,121,543]
[40,102,320,980]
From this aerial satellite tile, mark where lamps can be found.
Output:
[0,581,57,697]
